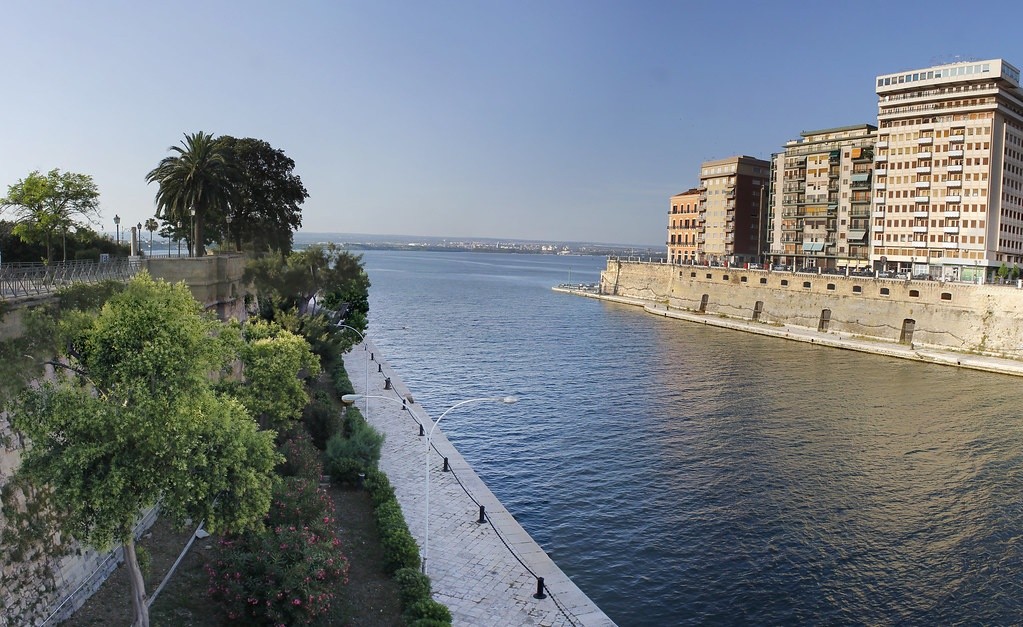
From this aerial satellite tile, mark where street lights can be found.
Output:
[167,226,172,255]
[187,202,195,256]
[226,213,232,256]
[114,214,120,245]
[177,218,183,258]
[137,222,142,254]
[339,395,518,572]
[337,322,411,430]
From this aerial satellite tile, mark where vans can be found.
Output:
[750,263,762,268]
[773,264,786,270]
[878,271,892,278]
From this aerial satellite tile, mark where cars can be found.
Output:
[934,275,954,282]
[895,272,907,279]
